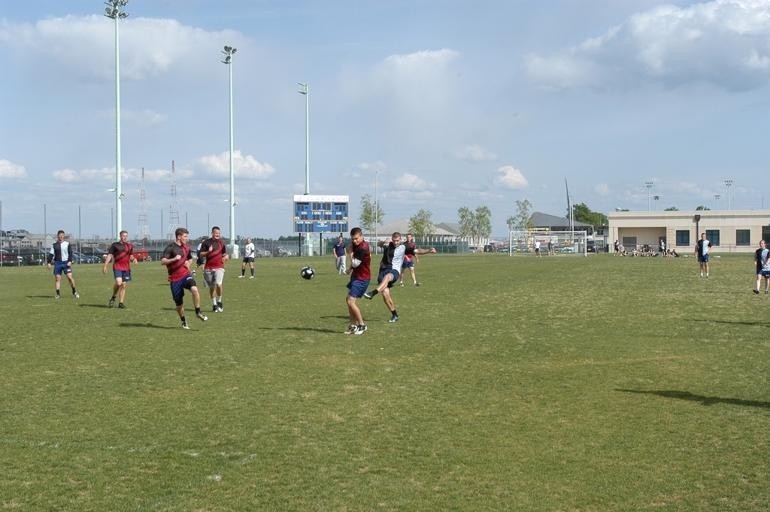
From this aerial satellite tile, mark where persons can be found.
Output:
[47,231,80,300]
[102,230,138,309]
[548,238,554,256]
[200,227,230,313]
[613,238,679,257]
[752,240,770,295]
[527,239,575,253]
[363,232,437,322]
[695,233,714,278]
[160,227,208,330]
[238,238,256,279]
[534,240,542,258]
[192,238,208,288]
[232,241,240,259]
[345,227,372,337]
[332,237,348,275]
[399,234,422,288]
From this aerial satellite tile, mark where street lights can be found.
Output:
[103,1,141,240]
[219,45,242,262]
[724,176,734,210]
[652,194,661,210]
[643,175,658,212]
[713,192,722,210]
[294,79,312,257]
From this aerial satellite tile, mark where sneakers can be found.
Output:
[386,315,400,323]
[118,303,128,309]
[54,294,61,299]
[363,292,374,300]
[238,274,246,279]
[414,282,421,286]
[248,275,256,280]
[195,312,209,322]
[212,300,224,314]
[343,324,368,335]
[71,290,81,299]
[109,298,115,309]
[400,280,405,287]
[181,320,189,330]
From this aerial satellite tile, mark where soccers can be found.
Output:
[301,267,314,279]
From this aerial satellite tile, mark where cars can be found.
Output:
[241,246,298,261]
[1,246,151,271]
[468,239,605,253]
